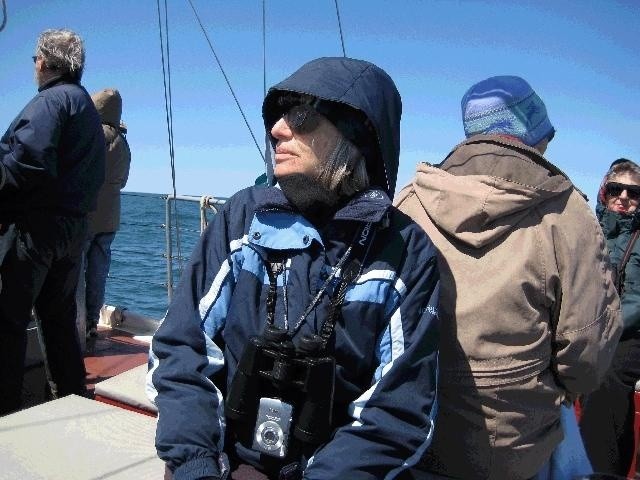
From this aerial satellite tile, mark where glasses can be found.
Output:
[32,55,41,62]
[266,103,319,134]
[605,182,640,201]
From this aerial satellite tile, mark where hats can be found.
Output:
[461,76,553,147]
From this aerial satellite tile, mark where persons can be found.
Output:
[390,75,624,480]
[148,58,441,480]
[83,89,131,339]
[0,29,106,415]
[579,157,640,480]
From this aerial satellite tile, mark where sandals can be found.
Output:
[85,323,98,346]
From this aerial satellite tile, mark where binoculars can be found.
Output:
[225,325,336,444]
